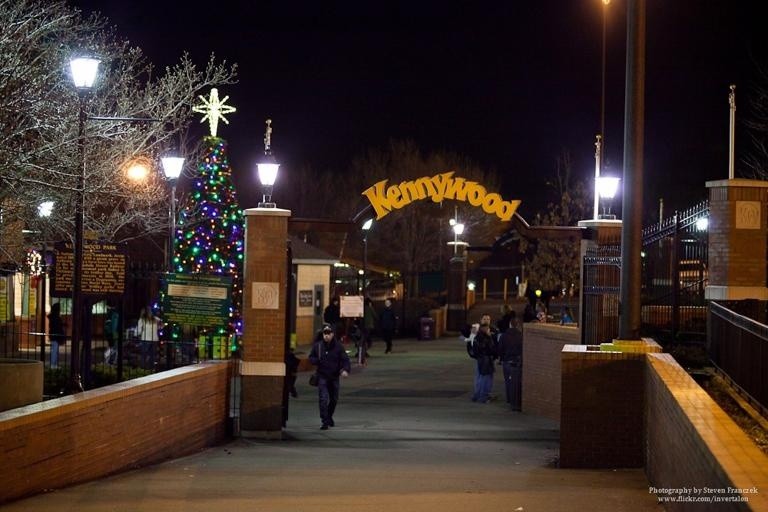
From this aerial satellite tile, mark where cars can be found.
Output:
[363,279,404,307]
[679,261,709,286]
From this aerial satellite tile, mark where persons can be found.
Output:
[133,304,165,370]
[378,298,399,353]
[308,325,351,429]
[364,297,379,356]
[101,305,127,374]
[47,302,66,369]
[323,296,348,342]
[460,300,573,411]
[348,317,369,369]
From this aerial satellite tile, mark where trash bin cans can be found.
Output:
[508,361,522,411]
[419,317,434,341]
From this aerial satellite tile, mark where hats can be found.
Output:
[322,326,332,333]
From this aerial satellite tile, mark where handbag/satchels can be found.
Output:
[309,374,319,386]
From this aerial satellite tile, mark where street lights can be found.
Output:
[160,137,185,362]
[59,45,106,392]
[360,217,373,267]
[36,200,54,368]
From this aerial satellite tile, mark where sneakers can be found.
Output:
[320,417,335,431]
